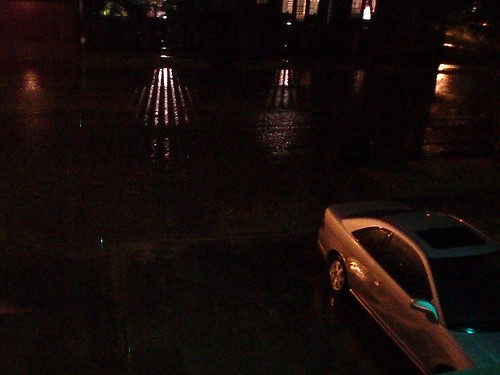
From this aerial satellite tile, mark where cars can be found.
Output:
[316,198,500,375]
